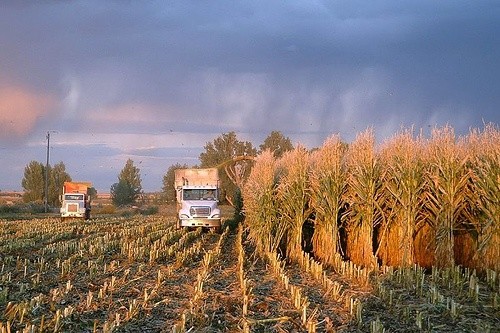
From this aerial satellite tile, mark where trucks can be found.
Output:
[59,182,92,220]
[174,168,221,233]
